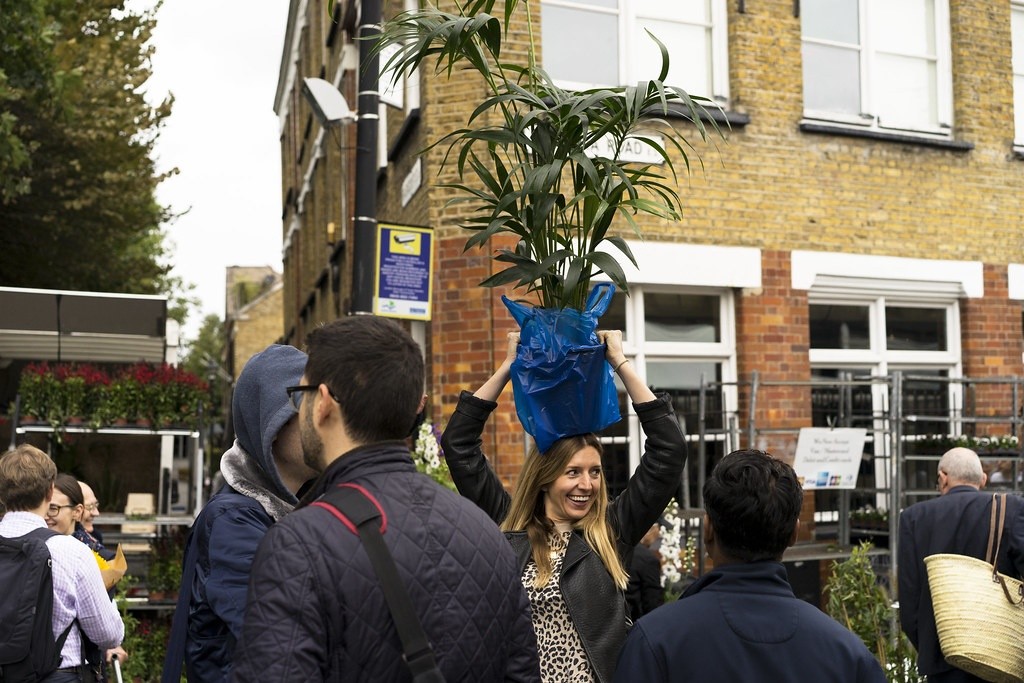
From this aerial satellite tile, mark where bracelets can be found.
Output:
[613,359,629,373]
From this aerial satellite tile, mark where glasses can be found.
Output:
[84,502,100,511]
[46,503,75,517]
[285,384,341,413]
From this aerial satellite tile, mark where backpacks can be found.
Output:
[0,527,64,683]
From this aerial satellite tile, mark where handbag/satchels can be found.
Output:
[923,492,1024,683]
[500,282,623,457]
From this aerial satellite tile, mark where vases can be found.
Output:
[64,417,81,424]
[161,420,171,429]
[20,417,35,424]
[149,592,164,600]
[134,418,151,425]
[111,416,128,425]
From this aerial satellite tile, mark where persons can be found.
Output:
[0,442,128,683]
[232,314,541,683]
[440,330,687,683]
[164,344,324,683]
[898,445,1024,683]
[614,449,885,683]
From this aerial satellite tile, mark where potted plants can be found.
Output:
[351,0,734,452]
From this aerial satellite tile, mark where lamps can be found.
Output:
[298,75,362,127]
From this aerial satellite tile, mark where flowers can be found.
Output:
[411,417,457,492]
[142,528,184,590]
[20,359,213,423]
[657,497,703,608]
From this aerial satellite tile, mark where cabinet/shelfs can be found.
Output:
[9,390,205,613]
[697,368,1024,654]
